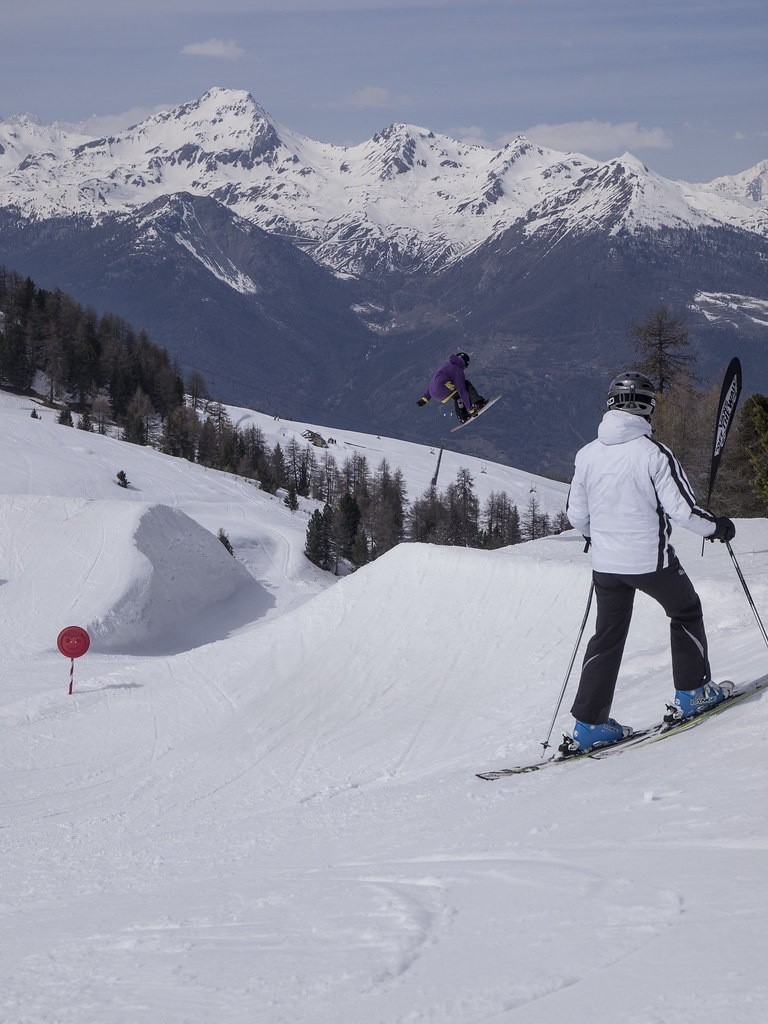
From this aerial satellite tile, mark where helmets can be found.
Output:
[606,372,656,416]
[457,353,469,367]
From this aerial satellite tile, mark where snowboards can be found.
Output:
[450,393,503,432]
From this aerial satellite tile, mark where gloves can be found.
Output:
[468,408,478,417]
[705,517,736,543]
[582,534,592,553]
[415,398,428,407]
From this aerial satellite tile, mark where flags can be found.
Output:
[700,356,744,557]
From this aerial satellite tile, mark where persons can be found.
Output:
[565,370,736,761]
[416,353,489,424]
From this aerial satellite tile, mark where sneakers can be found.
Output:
[473,397,489,410]
[674,680,734,717]
[572,719,634,751]
[458,411,470,424]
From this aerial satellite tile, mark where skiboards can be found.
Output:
[475,670,767,780]
